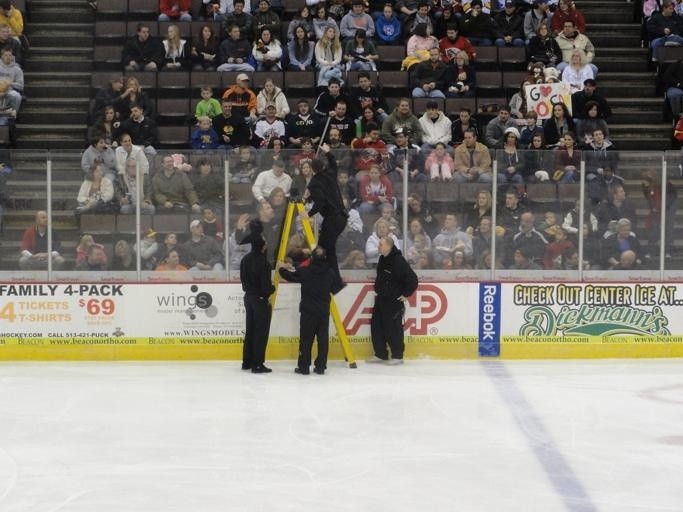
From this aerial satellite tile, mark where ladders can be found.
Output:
[269,196,358,370]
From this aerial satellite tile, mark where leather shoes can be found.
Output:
[313,365,325,374]
[294,366,310,374]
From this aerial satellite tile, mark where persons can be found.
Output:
[238,235,275,374]
[362,235,418,365]
[296,142,348,296]
[277,246,337,375]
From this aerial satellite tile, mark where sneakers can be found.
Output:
[252,363,272,373]
[384,357,405,366]
[241,362,253,370]
[365,355,389,363]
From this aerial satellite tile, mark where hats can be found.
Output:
[190,219,204,231]
[505,0,515,6]
[392,126,410,135]
[236,73,251,81]
[264,100,277,109]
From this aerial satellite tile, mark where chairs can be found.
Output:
[80,1,627,269]
[1,0,25,165]
[641,1,683,148]
